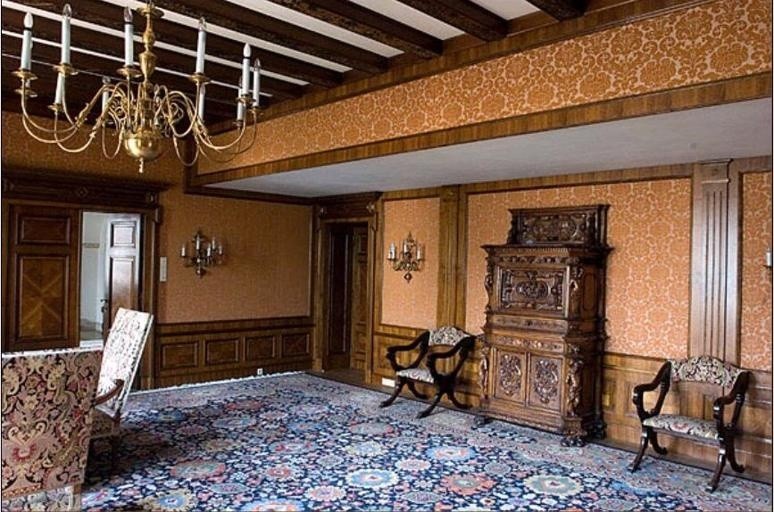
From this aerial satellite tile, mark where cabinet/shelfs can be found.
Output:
[474,243,616,447]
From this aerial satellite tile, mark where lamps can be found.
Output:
[385,231,426,284]
[11,0,261,173]
[178,226,227,279]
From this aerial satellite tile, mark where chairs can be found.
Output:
[627,355,753,493]
[1,307,154,512]
[378,323,477,419]
